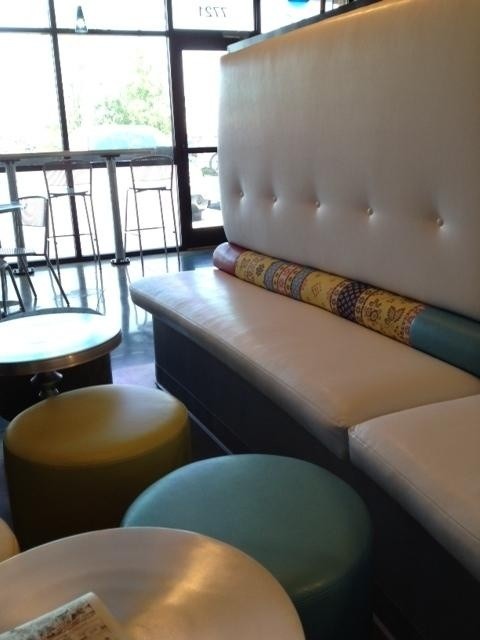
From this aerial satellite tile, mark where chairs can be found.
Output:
[42,157,101,271]
[1,195,71,318]
[124,154,180,265]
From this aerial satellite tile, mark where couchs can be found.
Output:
[126,0,480,640]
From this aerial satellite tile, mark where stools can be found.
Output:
[2,381,192,548]
[0,313,122,424]
[1,515,21,564]
[0,524,307,640]
[125,452,376,638]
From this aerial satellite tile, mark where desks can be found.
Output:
[0,203,30,317]
